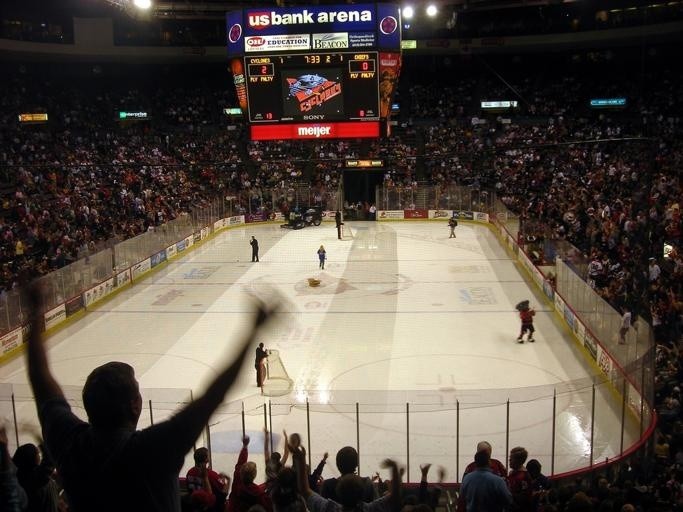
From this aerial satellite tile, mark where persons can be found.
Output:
[243,407,683,512]
[447,217,456,238]
[249,236,259,262]
[514,217,683,408]
[17,253,279,511]
[253,342,271,387]
[0,214,244,512]
[1,0,681,216]
[317,245,326,269]
